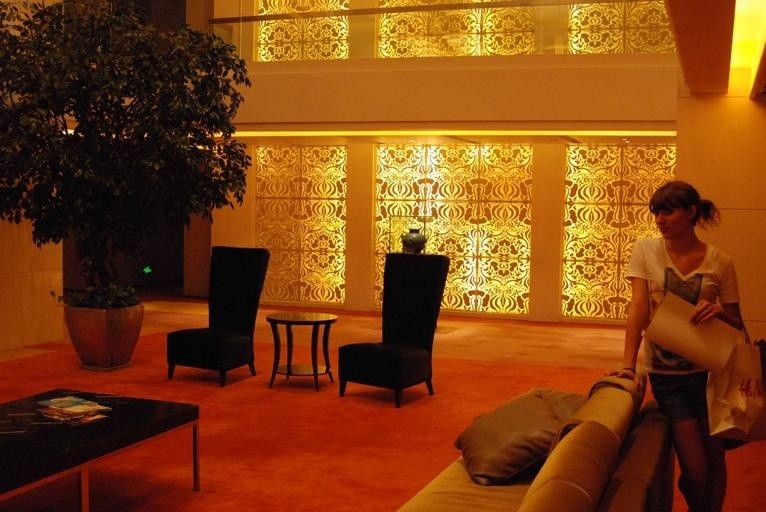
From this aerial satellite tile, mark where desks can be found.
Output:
[262,311,338,393]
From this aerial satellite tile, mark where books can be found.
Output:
[34,393,114,429]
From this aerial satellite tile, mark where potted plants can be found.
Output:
[0,0,253,367]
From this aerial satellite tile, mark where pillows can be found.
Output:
[532,383,589,426]
[454,387,578,487]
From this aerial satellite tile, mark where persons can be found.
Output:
[604,179,743,512]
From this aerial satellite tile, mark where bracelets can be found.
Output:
[622,366,636,373]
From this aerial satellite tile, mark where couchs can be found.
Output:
[399,370,673,512]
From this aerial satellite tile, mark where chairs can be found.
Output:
[170,245,270,383]
[337,255,450,408]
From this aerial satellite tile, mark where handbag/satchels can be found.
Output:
[705,323,765,441]
